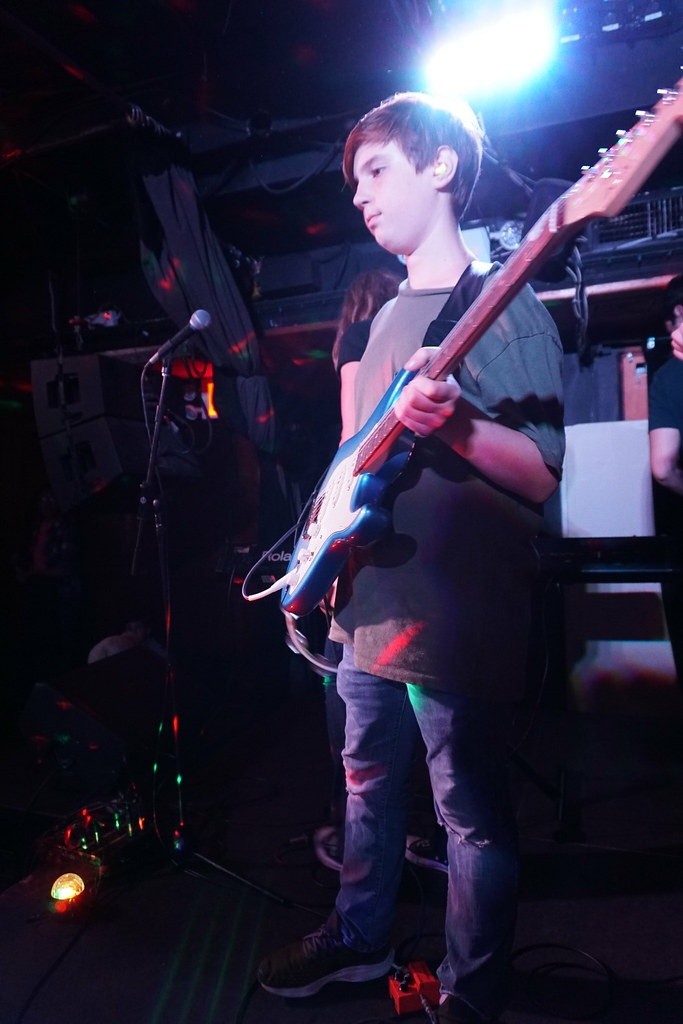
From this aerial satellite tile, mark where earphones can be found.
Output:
[433,163,446,176]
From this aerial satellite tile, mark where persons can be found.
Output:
[649,273,683,697]
[257,90,566,1024]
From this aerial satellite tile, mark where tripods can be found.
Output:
[73,353,293,938]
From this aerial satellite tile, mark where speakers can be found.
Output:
[30,350,206,521]
[521,178,579,284]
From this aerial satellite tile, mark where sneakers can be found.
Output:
[315,826,348,870]
[257,910,395,998]
[436,993,484,1024]
[403,832,450,875]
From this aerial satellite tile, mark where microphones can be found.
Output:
[145,309,211,370]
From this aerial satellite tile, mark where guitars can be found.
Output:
[278,78,683,619]
